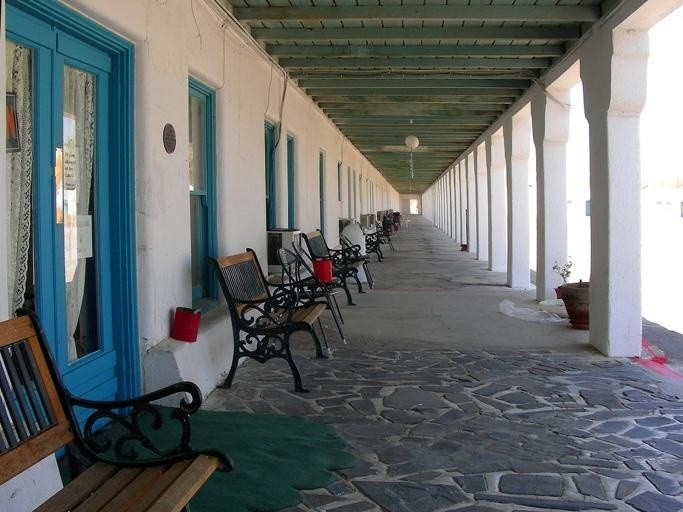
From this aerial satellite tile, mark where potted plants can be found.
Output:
[551,256,576,299]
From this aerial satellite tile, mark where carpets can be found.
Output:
[57,405,359,511]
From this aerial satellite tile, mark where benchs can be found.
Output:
[209,216,395,394]
[0,303,235,512]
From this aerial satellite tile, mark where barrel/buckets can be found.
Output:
[315,259,333,285]
[173,306,202,341]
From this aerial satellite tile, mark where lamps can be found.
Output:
[405,136,419,180]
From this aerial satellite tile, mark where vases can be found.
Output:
[559,281,591,324]
